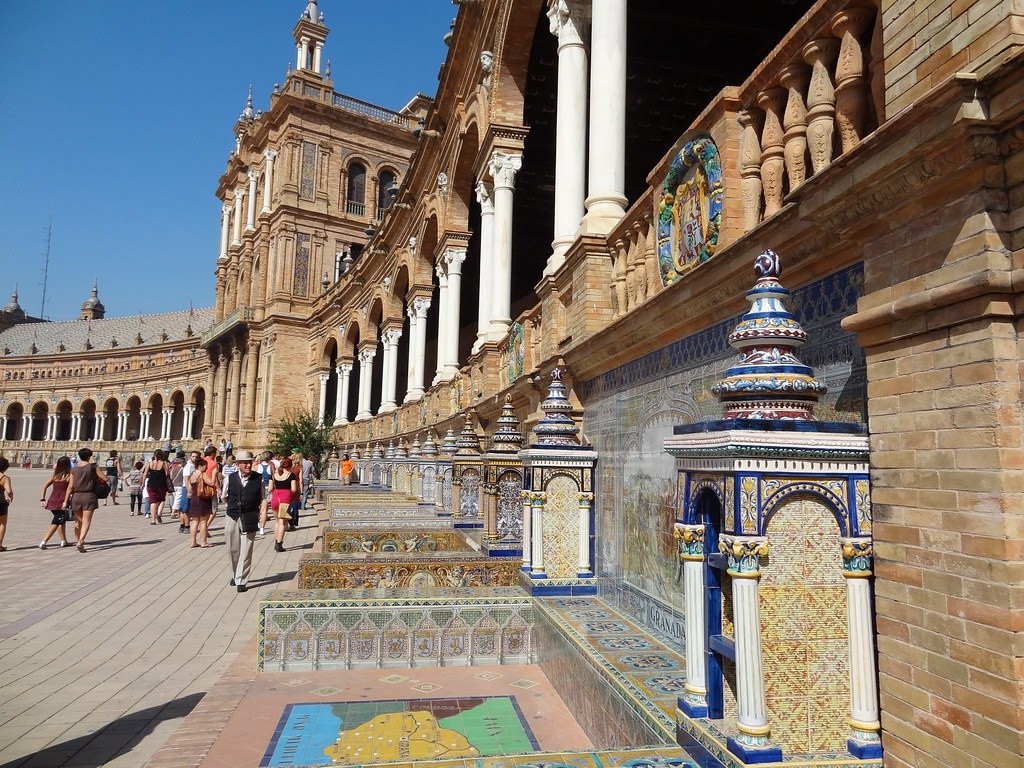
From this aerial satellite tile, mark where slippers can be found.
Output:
[190,543,200,548]
[201,543,215,547]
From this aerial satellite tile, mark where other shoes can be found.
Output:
[138,511,143,515]
[274,540,286,552]
[145,514,150,519]
[61,540,72,547]
[156,514,162,523]
[171,513,178,519]
[113,502,119,505]
[130,512,134,516]
[75,543,87,553]
[237,584,245,592]
[290,525,297,531]
[178,524,190,533]
[39,541,47,550]
[0,545,7,551]
[260,527,264,536]
[230,577,236,586]
[151,521,156,524]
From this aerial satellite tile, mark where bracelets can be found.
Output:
[41,499,45,501]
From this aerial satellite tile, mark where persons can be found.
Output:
[21,454,31,470]
[341,454,354,486]
[93,450,100,465]
[70,455,77,468]
[149,434,155,441]
[38,456,74,550]
[225,438,233,455]
[219,438,226,455]
[103,449,122,505]
[71,448,109,553]
[222,451,263,591]
[203,439,213,453]
[164,439,172,457]
[0,456,13,551]
[175,440,183,451]
[123,446,320,551]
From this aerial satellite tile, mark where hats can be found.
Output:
[232,451,255,461]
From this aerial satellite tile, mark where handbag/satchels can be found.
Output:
[90,463,110,498]
[278,502,293,520]
[197,472,216,498]
[118,478,123,491]
